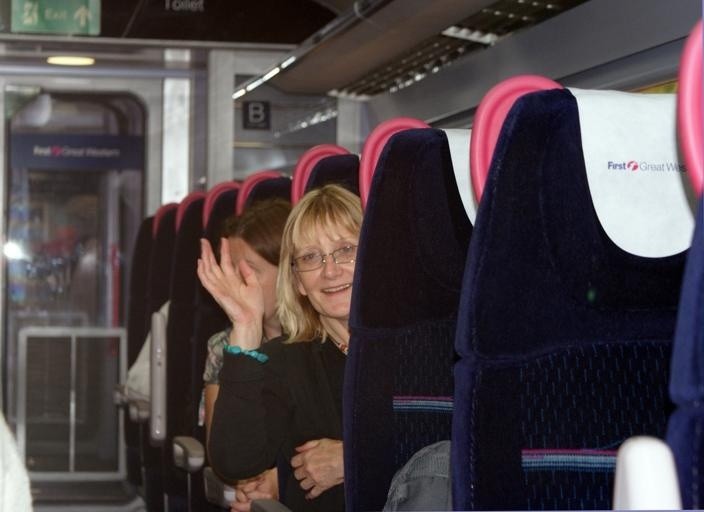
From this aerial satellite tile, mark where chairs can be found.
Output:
[127,16,704,512]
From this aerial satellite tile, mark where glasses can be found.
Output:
[293,245,358,272]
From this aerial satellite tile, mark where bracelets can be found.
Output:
[223,343,269,363]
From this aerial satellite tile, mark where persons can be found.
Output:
[197,197,294,512]
[196,182,364,512]
[122,300,169,422]
